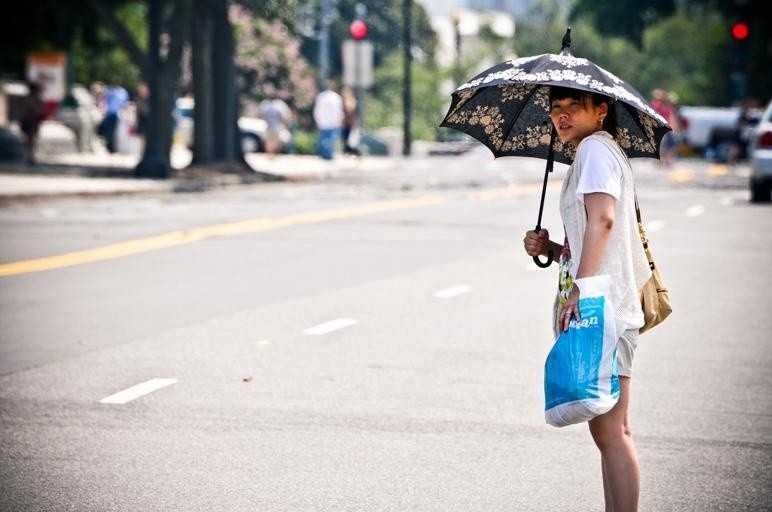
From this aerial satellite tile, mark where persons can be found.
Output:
[651,87,761,168]
[313,78,363,161]
[17,76,294,168]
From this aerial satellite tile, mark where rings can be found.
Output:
[523,86,655,512]
[566,312,571,314]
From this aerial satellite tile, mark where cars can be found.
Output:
[746,97,772,201]
[114,95,271,157]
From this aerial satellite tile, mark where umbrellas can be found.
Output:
[437,25,673,268]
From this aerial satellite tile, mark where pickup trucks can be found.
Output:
[675,105,749,164]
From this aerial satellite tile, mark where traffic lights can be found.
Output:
[729,18,749,55]
[347,1,369,42]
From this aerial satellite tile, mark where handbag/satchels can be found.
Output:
[638,242,672,336]
